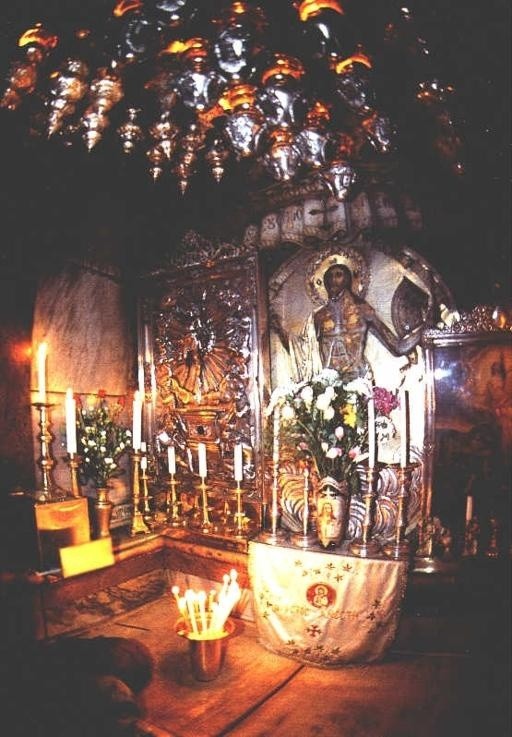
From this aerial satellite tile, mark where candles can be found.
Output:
[271,408,280,464]
[367,399,377,468]
[399,386,408,468]
[39,341,48,401]
[140,441,148,469]
[132,391,143,450]
[168,446,176,474]
[169,569,242,635]
[64,388,78,459]
[198,444,209,478]
[465,496,473,522]
[234,444,244,481]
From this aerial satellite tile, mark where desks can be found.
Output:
[248,531,413,670]
[36,495,91,571]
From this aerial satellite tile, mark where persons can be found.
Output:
[271,265,432,385]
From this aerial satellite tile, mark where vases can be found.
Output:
[94,484,116,536]
[315,479,348,552]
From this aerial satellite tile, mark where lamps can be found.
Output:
[0,0,493,197]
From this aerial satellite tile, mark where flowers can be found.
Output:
[283,374,398,485]
[76,391,131,485]
